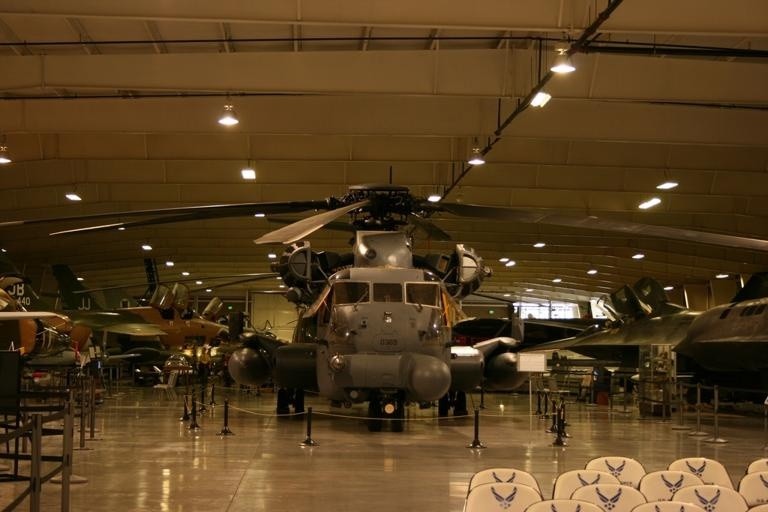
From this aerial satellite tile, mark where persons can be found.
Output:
[199,346,210,392]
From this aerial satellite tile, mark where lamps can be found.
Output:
[549,42,576,74]
[468,141,486,165]
[218,97,239,126]
[0,135,13,163]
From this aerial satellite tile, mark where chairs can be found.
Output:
[152,370,179,401]
[463,456,768,512]
